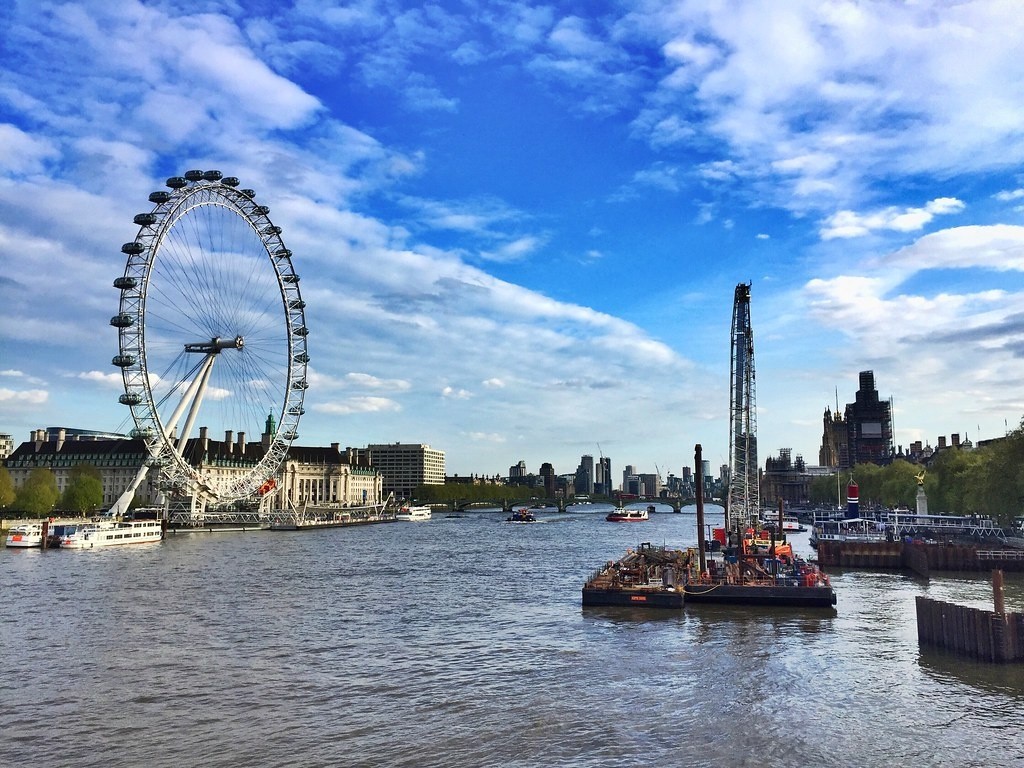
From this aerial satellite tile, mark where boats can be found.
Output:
[580,513,838,611]
[605,504,649,522]
[647,504,656,514]
[396,507,431,522]
[60,519,162,549]
[501,507,544,523]
[4,520,90,548]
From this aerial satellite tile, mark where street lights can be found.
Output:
[51,504,55,517]
[3,505,5,519]
[92,504,96,522]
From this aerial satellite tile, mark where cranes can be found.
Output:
[724,277,760,541]
